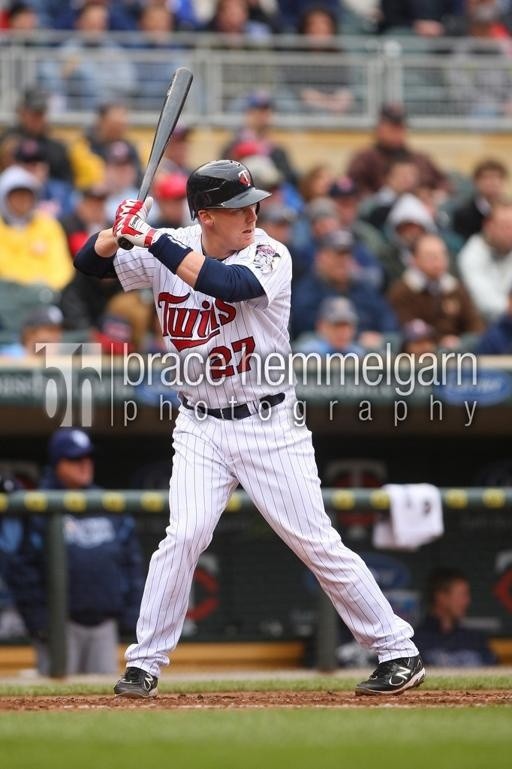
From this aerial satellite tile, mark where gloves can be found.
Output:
[113,197,163,246]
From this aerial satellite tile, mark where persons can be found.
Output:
[0,0,511,697]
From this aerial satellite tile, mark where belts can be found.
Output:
[181,392,285,419]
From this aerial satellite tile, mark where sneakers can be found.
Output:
[356,653,426,695]
[114,666,158,697]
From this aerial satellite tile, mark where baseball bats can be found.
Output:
[118,66,194,251]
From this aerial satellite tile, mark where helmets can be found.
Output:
[185,159,272,221]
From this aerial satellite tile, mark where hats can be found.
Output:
[49,429,94,464]
